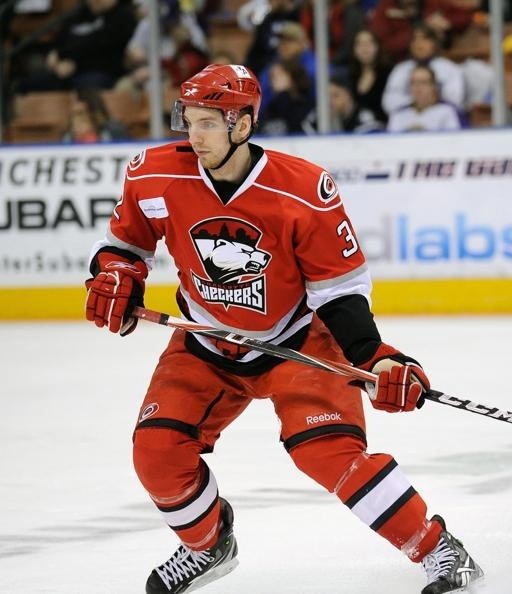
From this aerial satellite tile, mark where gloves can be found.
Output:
[352,342,430,413]
[84,252,148,337]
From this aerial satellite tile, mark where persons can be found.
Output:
[86,64,485,594]
[0,1,511,145]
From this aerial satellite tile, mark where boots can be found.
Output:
[420,515,484,594]
[145,497,238,594]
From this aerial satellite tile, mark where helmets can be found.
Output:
[180,63,262,125]
